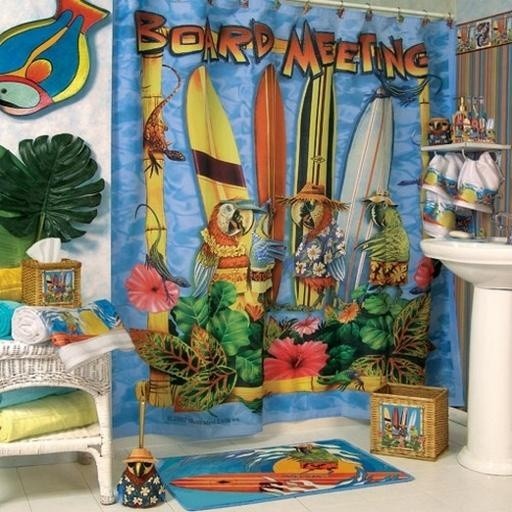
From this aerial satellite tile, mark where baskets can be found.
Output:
[21,258,83,309]
[369,380,451,463]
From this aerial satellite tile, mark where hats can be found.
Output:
[361,191,400,208]
[273,183,351,212]
[220,199,267,215]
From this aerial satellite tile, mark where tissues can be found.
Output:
[20,237,83,308]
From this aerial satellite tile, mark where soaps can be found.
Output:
[449,229,470,239]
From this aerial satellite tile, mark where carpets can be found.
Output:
[151,436,415,512]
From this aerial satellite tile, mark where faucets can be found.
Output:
[492,209,512,242]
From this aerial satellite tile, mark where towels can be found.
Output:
[1,295,136,374]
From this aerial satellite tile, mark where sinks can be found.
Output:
[420,237,512,269]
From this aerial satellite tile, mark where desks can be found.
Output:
[1,338,117,505]
